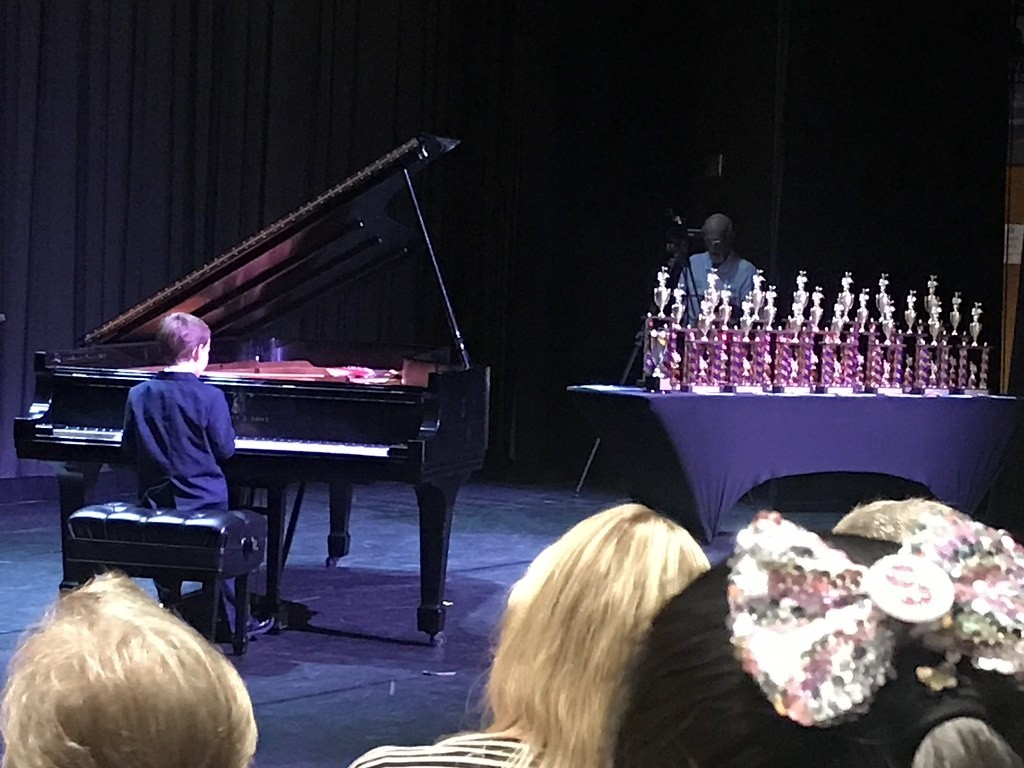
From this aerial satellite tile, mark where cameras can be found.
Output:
[667,216,704,246]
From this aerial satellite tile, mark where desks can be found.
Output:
[561,383,1022,547]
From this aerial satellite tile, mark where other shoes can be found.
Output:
[231,612,275,644]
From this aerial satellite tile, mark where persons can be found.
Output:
[0,568,260,768]
[676,211,759,325]
[350,501,1024,768]
[121,312,275,641]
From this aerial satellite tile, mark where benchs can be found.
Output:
[61,502,268,656]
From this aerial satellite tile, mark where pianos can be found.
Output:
[11,126,492,649]
